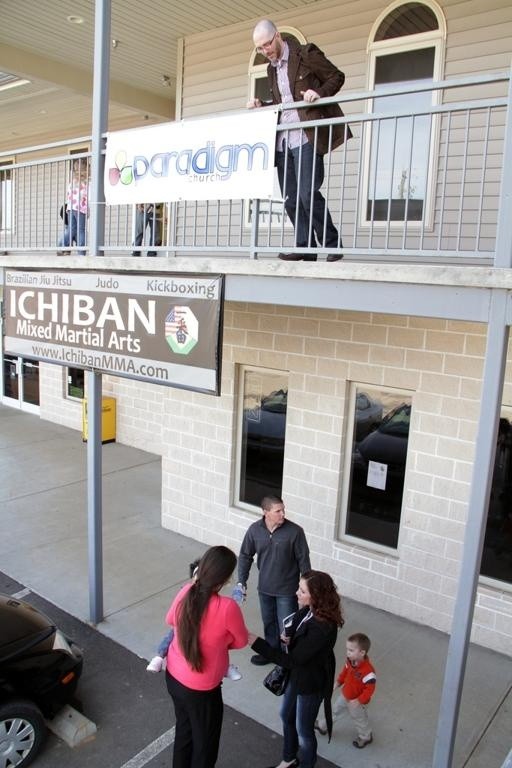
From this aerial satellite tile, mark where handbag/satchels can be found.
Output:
[60,204,67,224]
[262,664,289,697]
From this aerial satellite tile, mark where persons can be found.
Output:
[229,492,313,666]
[314,631,378,749]
[246,18,355,263]
[163,544,248,766]
[146,557,243,682]
[55,148,90,254]
[130,203,165,256]
[247,568,344,766]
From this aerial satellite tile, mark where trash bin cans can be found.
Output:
[83,397,116,444]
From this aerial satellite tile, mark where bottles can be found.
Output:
[232,583,243,606]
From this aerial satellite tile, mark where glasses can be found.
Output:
[255,32,276,54]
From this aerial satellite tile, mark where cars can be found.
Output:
[1,594,84,768]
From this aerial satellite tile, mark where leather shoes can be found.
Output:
[279,246,318,260]
[327,243,343,261]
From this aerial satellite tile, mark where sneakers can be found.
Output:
[131,250,141,256]
[251,651,274,665]
[147,656,163,674]
[148,251,157,256]
[353,733,373,748]
[56,250,72,256]
[224,665,241,681]
[314,720,327,736]
